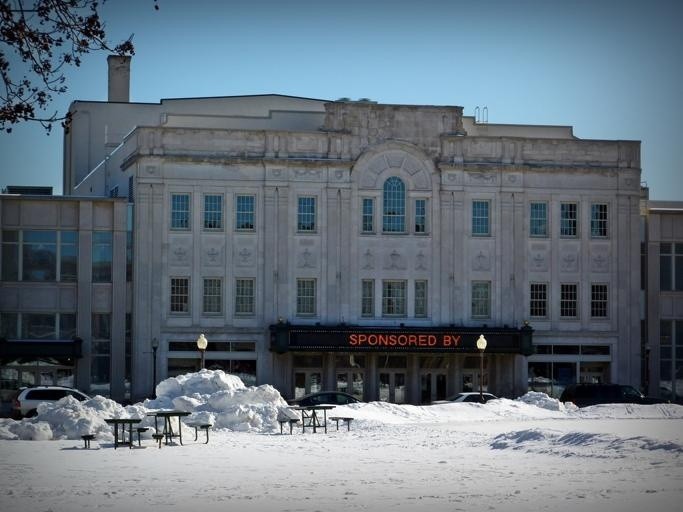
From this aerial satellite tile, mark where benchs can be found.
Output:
[329,416,354,430]
[81,434,95,449]
[187,421,212,444]
[152,434,163,449]
[125,427,149,446]
[279,419,300,435]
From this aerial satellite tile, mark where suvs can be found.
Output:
[557,382,672,408]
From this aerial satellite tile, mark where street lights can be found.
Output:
[195,331,208,371]
[150,335,159,401]
[475,331,490,402]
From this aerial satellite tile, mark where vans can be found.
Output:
[8,384,92,420]
[88,379,131,399]
[0,379,32,402]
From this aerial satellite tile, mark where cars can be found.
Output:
[430,392,500,405]
[286,389,362,407]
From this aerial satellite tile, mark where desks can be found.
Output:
[286,405,336,433]
[147,410,192,446]
[104,418,142,449]
